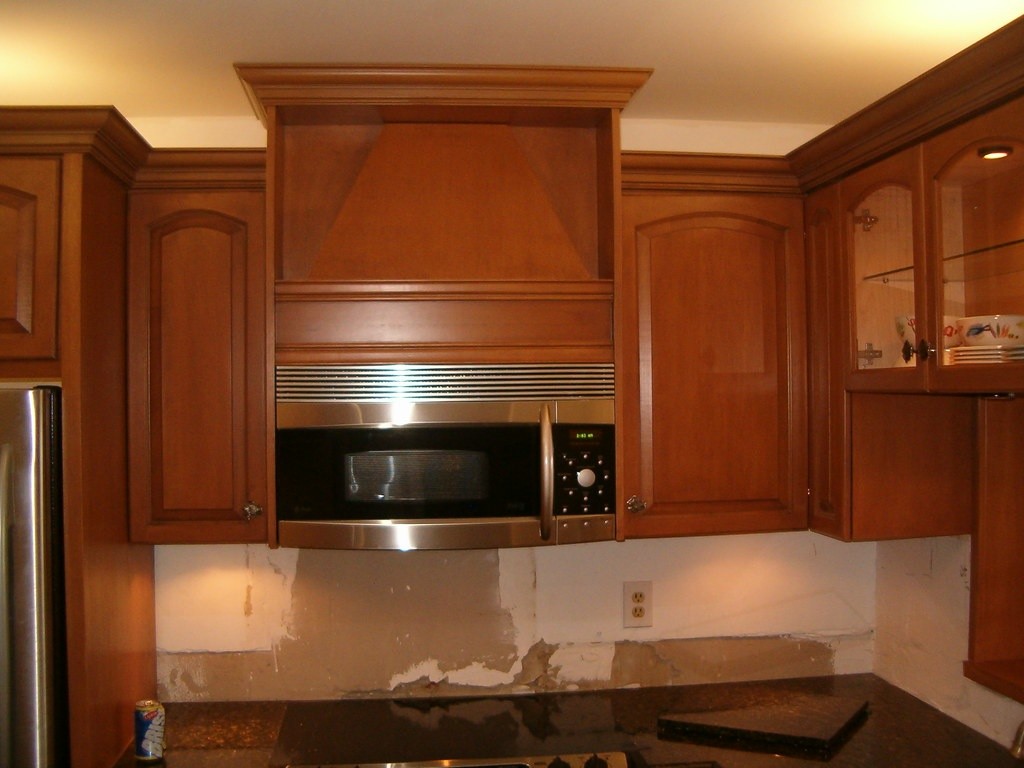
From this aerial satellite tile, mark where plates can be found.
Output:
[950,345,1024,364]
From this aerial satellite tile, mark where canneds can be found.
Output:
[135,699,166,760]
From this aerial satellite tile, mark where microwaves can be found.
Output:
[276,363,616,551]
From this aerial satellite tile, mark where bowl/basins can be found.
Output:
[896,315,962,353]
[957,314,1024,346]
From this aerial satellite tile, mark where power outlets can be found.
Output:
[622,580,653,629]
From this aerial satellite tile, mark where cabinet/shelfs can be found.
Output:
[0,155,59,360]
[840,95,1024,397]
[623,190,810,539]
[805,183,852,543]
[128,187,270,546]
